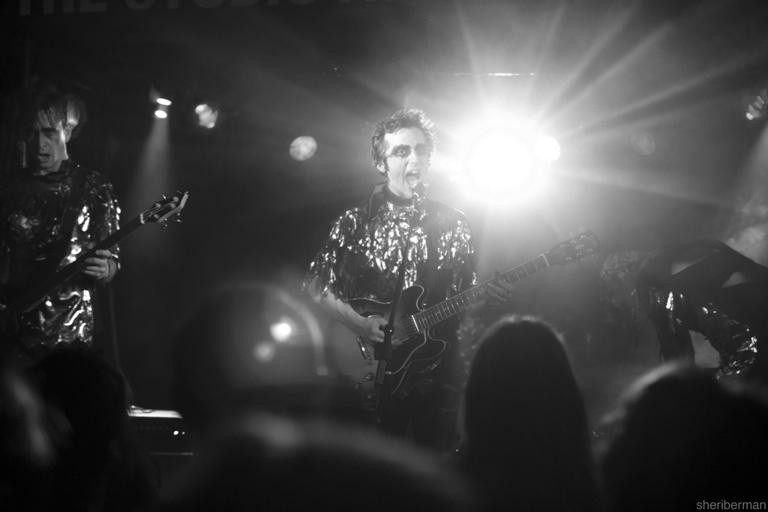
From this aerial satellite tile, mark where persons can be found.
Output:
[0,87,121,348]
[296,110,520,450]
[597,238,768,379]
[0,316,768,512]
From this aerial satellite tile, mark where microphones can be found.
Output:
[16,141,27,170]
[412,181,427,201]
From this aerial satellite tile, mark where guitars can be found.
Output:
[349,230,603,410]
[3,189,188,355]
